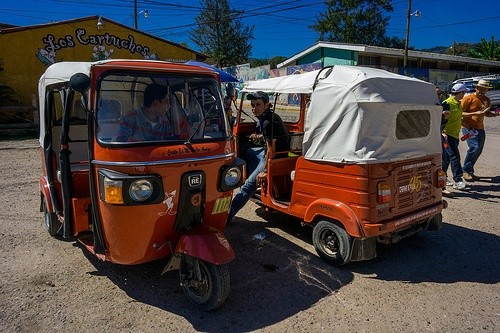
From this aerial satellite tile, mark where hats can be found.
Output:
[451,83,469,93]
[246,91,269,102]
[473,79,493,88]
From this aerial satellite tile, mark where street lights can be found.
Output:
[133,9,149,29]
[403,10,421,77]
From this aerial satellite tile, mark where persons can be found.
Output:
[436,79,492,194]
[225,91,289,224]
[118,84,176,142]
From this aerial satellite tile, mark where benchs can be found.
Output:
[262,150,302,179]
[52,119,126,181]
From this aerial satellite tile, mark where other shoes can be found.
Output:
[454,181,467,190]
[446,178,455,186]
[471,174,480,180]
[461,172,472,180]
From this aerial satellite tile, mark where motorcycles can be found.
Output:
[36,59,247,311]
[453,73,500,112]
[231,66,448,267]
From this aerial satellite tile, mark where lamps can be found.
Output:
[97,15,106,30]
[143,8,148,17]
